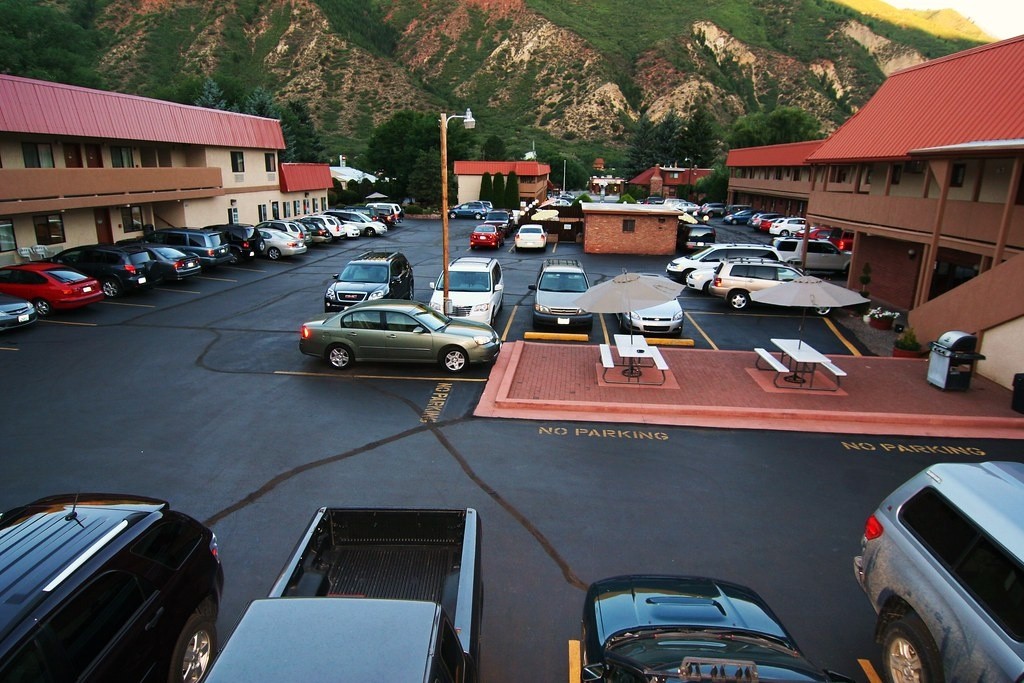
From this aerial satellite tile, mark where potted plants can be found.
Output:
[893,327,922,358]
[855,261,871,314]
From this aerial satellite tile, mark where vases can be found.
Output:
[863,315,871,324]
[869,317,894,329]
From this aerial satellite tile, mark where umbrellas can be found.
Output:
[749,271,871,349]
[572,268,686,344]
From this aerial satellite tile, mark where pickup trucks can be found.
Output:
[484,210,516,237]
[199,505,485,683]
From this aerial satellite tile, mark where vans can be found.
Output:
[852,460,1024,683]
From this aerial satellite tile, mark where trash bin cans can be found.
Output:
[1011,373,1024,414]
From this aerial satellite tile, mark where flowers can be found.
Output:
[867,306,901,321]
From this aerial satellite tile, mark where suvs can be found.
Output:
[137,223,265,267]
[324,251,415,313]
[0,492,226,683]
[322,202,405,236]
[664,221,834,317]
[767,236,853,272]
[428,256,505,329]
[528,258,593,331]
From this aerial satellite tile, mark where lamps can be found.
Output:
[908,249,916,260]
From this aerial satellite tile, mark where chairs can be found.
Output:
[18,245,56,262]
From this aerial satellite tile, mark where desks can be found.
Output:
[614,334,654,383]
[770,338,832,388]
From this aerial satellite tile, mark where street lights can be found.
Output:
[684,157,691,185]
[439,108,476,318]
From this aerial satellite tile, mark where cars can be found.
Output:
[251,214,361,259]
[613,272,684,336]
[514,223,550,250]
[447,201,495,220]
[549,192,575,206]
[581,574,857,683]
[0,239,202,331]
[300,299,503,373]
[469,224,506,250]
[643,196,854,252]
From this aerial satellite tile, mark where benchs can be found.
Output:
[598,344,669,386]
[754,348,848,391]
